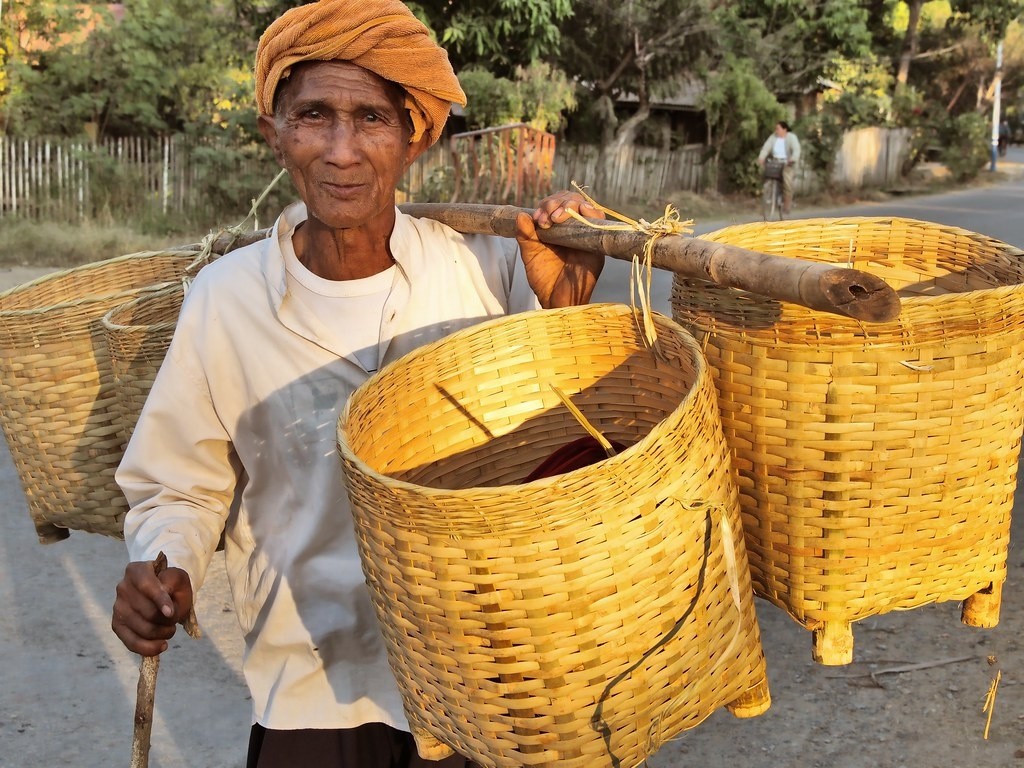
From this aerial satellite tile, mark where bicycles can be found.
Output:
[756,156,794,223]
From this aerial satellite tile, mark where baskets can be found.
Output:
[338,303,772,768]
[0,243,227,550]
[673,216,1024,666]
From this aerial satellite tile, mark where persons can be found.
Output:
[110,0,608,768]
[757,121,801,214]
